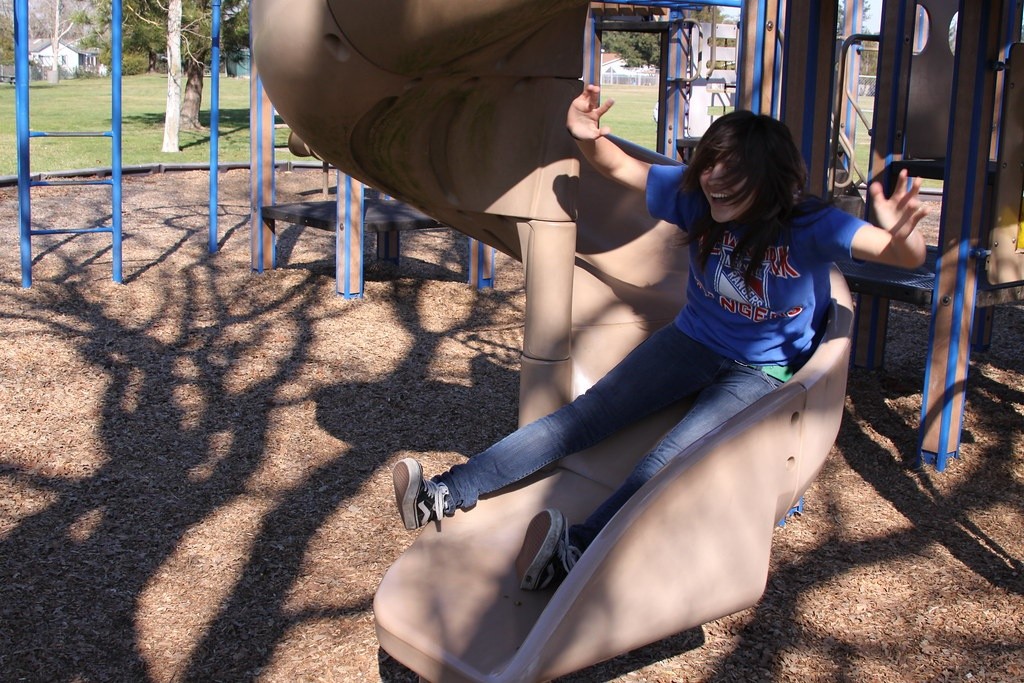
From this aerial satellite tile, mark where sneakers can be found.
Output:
[515,508,582,591]
[393,458,450,530]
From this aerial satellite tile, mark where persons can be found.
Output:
[652,85,688,138]
[392,84,927,590]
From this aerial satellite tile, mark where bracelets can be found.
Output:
[567,129,583,141]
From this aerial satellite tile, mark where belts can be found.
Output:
[735,360,794,383]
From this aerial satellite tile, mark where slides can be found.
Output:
[254,2,854,683]
